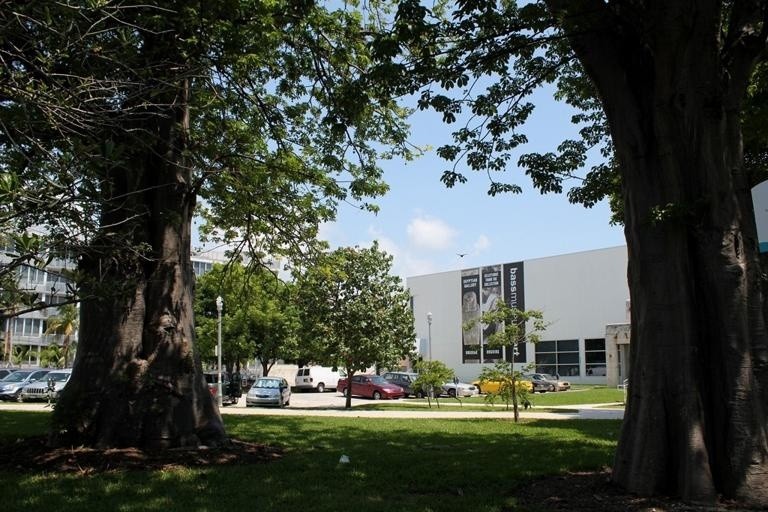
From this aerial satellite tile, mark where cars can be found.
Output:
[246,377,291,408]
[337,374,405,399]
[523,373,570,392]
[472,375,534,394]
[0,368,73,402]
[442,382,479,398]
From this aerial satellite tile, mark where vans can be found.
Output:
[296,365,348,391]
[380,372,426,399]
[203,371,225,396]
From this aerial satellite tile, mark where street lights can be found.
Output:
[427,313,434,399]
[216,296,223,407]
